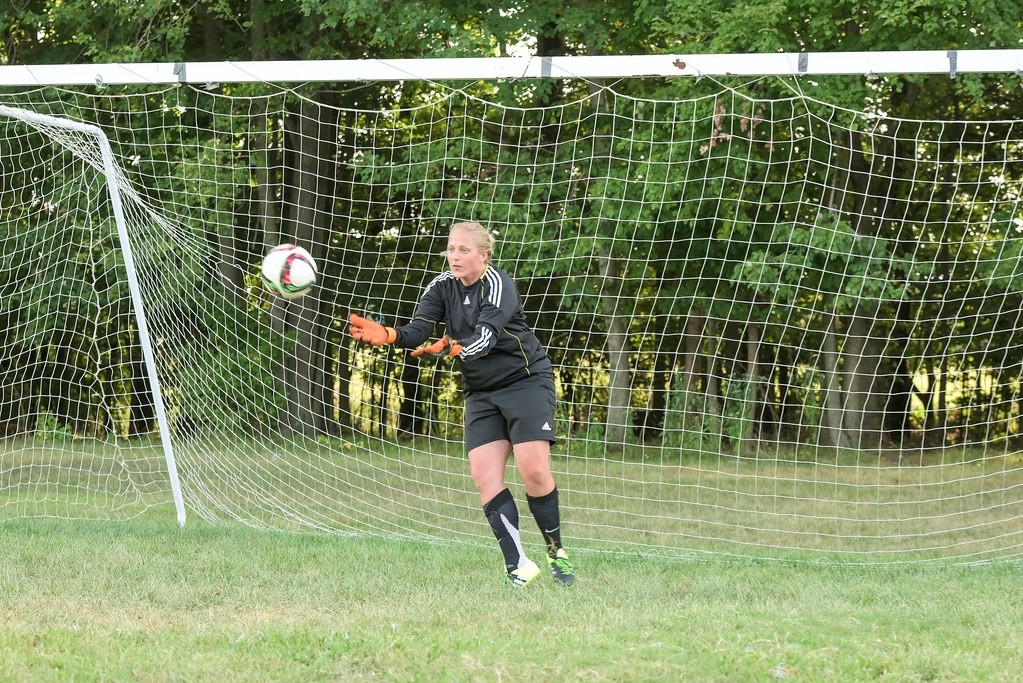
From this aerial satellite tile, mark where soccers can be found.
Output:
[259,242,320,301]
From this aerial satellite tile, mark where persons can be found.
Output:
[348,221,576,590]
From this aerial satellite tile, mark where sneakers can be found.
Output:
[504,557,541,590]
[546,548,575,587]
